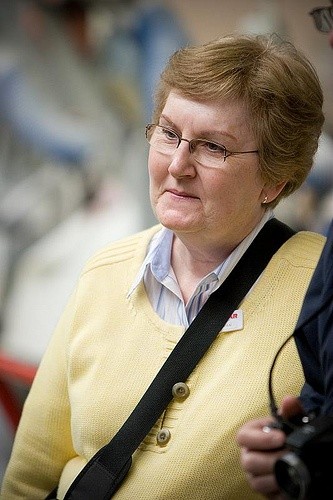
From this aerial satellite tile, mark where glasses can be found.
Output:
[145,123,258,168]
[309,7,333,33]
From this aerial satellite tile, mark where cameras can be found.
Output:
[262,411,333,500]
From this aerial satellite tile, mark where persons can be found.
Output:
[1,31,327,500]
[236,393,305,500]
